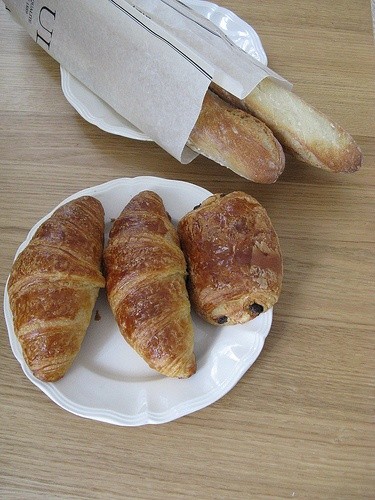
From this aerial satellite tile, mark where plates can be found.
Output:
[60,0,267,142]
[4,176,273,426]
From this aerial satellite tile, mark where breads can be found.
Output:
[184,86,285,184]
[178,190,283,326]
[104,190,197,380]
[244,78,363,174]
[7,195,106,383]
